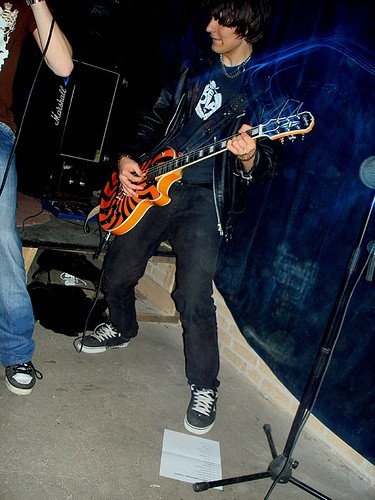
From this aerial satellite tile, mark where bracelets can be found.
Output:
[26,0,44,7]
[238,150,256,161]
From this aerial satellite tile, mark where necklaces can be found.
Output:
[220,53,251,78]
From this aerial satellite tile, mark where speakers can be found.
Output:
[38,58,120,165]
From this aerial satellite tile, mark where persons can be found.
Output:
[78,0,276,434]
[0,0,73,395]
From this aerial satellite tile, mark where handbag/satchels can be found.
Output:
[27,246,109,338]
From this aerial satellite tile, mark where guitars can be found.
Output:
[99,110,314,236]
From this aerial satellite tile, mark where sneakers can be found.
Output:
[183,382,218,434]
[76,322,130,353]
[4,359,41,396]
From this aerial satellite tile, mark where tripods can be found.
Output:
[189,189,374,500]
